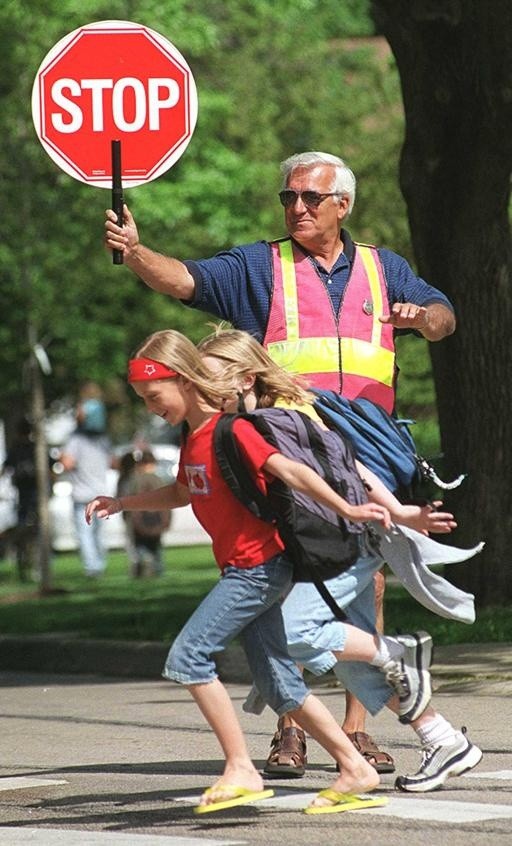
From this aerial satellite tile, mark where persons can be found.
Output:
[77,327,402,823]
[101,146,459,777]
[194,319,485,797]
[1,372,177,595]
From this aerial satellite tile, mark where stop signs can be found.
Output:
[31,14,202,195]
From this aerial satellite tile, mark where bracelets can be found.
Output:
[112,492,125,513]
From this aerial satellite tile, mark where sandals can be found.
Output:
[336,732,394,772]
[264,727,307,774]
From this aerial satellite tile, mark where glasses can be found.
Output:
[279,191,338,207]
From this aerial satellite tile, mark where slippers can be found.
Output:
[303,791,384,814]
[193,787,274,815]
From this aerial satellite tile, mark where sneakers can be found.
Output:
[379,630,433,723]
[396,727,482,791]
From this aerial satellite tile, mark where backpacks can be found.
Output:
[213,408,367,580]
[256,389,435,507]
